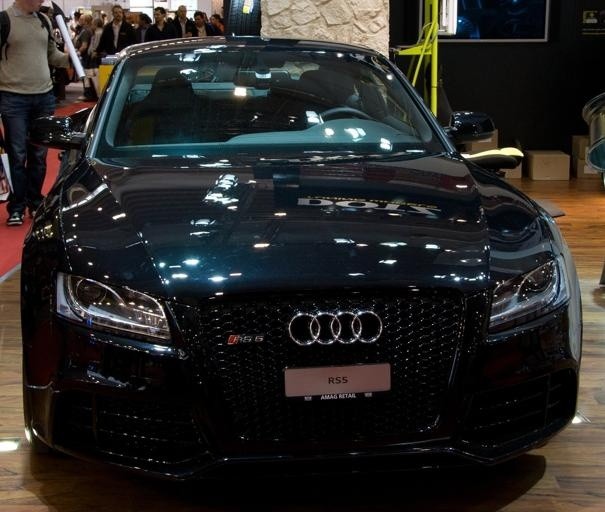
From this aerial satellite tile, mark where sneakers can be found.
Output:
[6,210,26,226]
[77,96,89,102]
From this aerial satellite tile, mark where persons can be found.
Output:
[0,0,83,227]
[38,4,226,107]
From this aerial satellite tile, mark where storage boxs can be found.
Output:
[468,128,602,180]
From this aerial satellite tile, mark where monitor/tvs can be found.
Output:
[418,0,551,43]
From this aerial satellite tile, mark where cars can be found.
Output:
[20,32,584,484]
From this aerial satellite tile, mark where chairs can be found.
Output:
[129,67,336,142]
